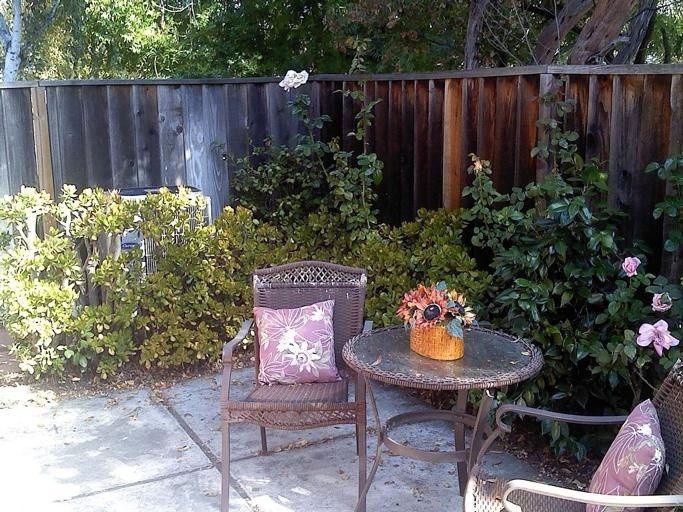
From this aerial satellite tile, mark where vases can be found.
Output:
[406,319,466,362]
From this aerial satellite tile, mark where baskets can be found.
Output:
[409,324,464,360]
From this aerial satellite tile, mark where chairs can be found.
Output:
[222,260,368,512]
[464,356,683,512]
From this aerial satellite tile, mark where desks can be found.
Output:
[340,322,545,512]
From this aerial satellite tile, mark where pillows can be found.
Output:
[252,299,343,387]
[584,397,666,512]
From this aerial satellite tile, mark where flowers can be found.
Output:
[395,280,476,338]
